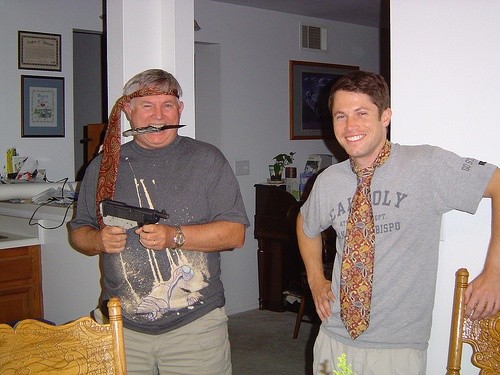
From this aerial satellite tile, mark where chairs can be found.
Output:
[445,268,500,375]
[0,297,127,375]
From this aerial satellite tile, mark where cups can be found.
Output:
[285,167,299,200]
[269,165,283,181]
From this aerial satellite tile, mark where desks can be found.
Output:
[0,201,102,325]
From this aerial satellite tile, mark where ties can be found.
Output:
[95,88,180,231]
[340,138,391,341]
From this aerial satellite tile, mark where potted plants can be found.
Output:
[269,152,296,181]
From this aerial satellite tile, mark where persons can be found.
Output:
[65,70,249,375]
[295,71,500,374]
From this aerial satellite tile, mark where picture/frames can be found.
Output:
[21,75,65,137]
[290,60,360,140]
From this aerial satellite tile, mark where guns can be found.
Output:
[98,197,170,232]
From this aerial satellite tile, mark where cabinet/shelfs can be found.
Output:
[0,230,44,327]
[254,183,337,314]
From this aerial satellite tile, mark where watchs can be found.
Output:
[169,226,187,250]
